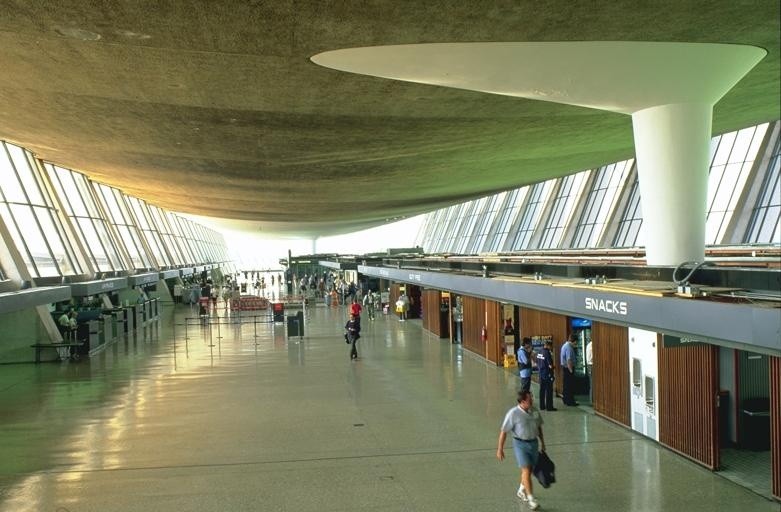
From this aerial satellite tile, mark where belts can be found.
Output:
[513,436,537,443]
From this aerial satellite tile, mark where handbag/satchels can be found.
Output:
[533,451,556,489]
[344,331,351,344]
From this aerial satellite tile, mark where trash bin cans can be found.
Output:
[743,398,769,452]
[324,295,332,306]
[273,303,283,325]
[286,312,303,340]
[240,284,245,293]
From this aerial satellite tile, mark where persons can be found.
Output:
[586,337,594,405]
[208,272,412,322]
[59,310,70,338]
[346,313,362,360]
[497,389,547,509]
[536,339,558,411]
[518,337,534,391]
[561,333,580,406]
[70,312,78,331]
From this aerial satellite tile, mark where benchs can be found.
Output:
[30,343,84,365]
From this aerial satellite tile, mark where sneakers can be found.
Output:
[516,483,540,510]
